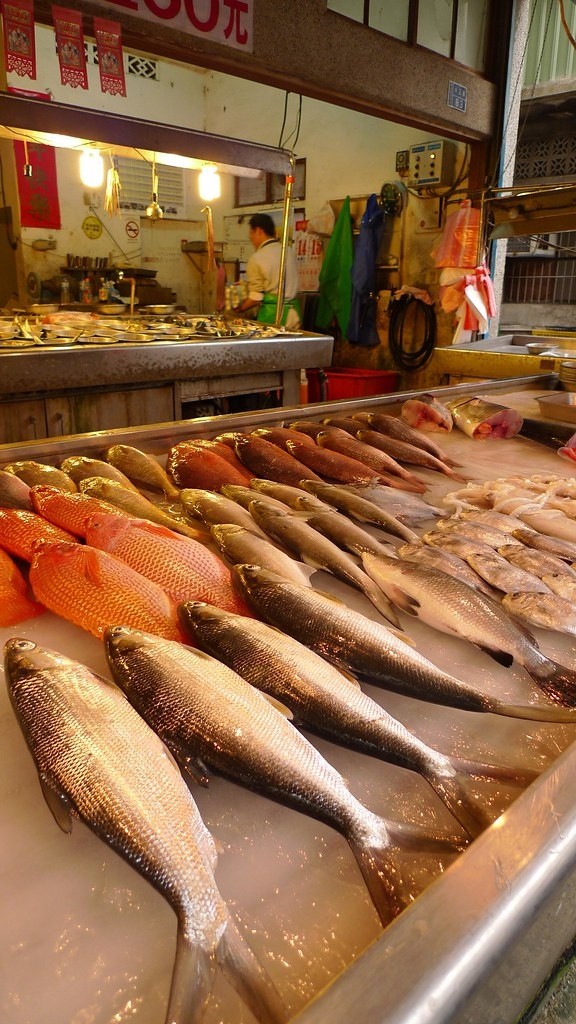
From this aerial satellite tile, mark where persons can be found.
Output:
[233,214,302,330]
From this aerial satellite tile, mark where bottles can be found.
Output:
[98,277,108,304]
[61,279,70,303]
[84,278,92,304]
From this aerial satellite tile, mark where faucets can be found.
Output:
[116,270,124,284]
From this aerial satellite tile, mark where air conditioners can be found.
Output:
[507,234,557,259]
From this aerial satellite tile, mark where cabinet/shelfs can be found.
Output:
[0,380,183,444]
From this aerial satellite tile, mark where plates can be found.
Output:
[0,318,303,348]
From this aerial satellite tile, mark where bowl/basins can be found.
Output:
[559,362,576,392]
[526,342,558,355]
[30,303,60,315]
[93,304,128,315]
[144,305,177,314]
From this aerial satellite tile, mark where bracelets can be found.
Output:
[239,307,244,313]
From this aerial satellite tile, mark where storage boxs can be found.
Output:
[534,391,576,424]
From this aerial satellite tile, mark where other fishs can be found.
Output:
[0,391,576,1022]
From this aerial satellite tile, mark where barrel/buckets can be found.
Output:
[322,367,399,402]
